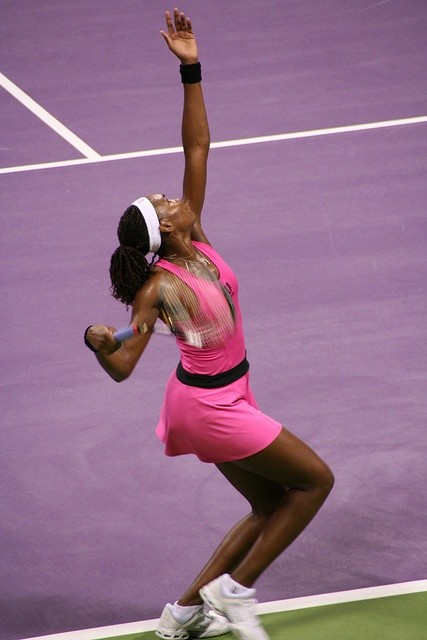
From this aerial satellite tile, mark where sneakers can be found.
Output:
[199,574,270,640]
[156,603,230,640]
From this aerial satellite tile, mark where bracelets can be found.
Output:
[83,324,93,351]
[179,62,202,84]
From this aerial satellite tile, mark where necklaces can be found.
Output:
[162,247,211,266]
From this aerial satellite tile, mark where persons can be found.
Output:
[83,6,334,640]
